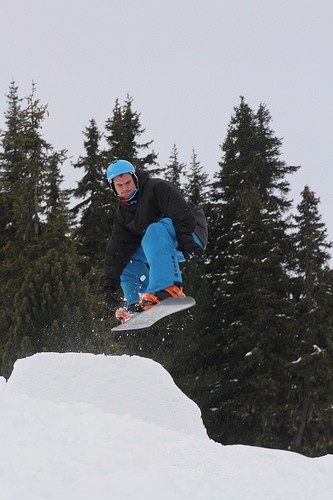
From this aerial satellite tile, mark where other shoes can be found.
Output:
[142,285,185,306]
[116,303,143,321]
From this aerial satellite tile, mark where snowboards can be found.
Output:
[110,296,196,331]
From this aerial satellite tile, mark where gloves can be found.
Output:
[177,234,206,263]
[103,288,121,307]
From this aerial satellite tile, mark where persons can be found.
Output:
[101,160,208,325]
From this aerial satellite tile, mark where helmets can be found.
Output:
[106,159,139,196]
